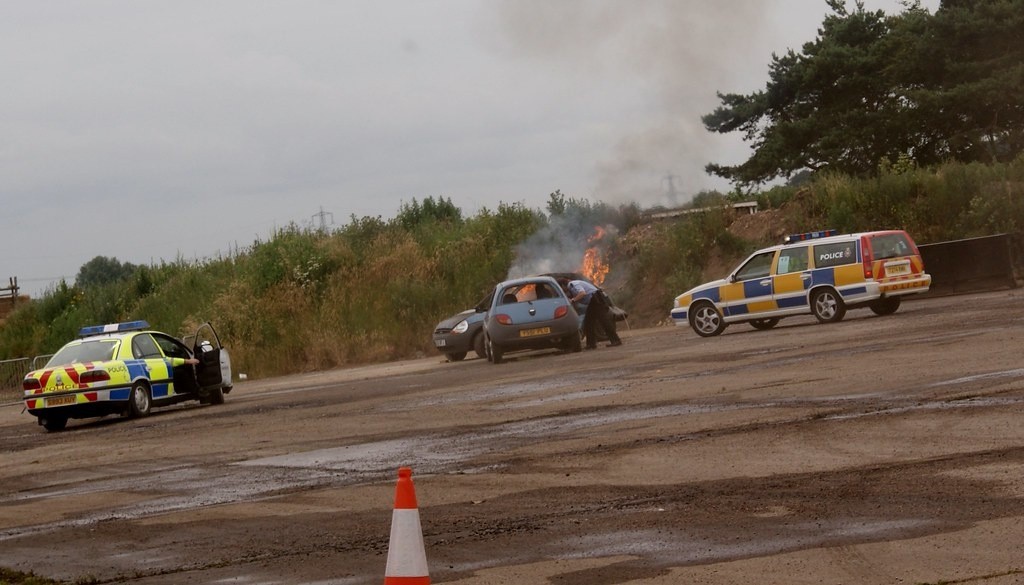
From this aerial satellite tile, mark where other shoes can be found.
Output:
[606,341,622,347]
[582,345,597,350]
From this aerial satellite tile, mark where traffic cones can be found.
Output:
[383,467,431,585]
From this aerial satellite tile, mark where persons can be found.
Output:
[557,278,621,349]
[158,343,210,401]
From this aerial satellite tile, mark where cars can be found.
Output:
[432,272,616,362]
[475,276,582,363]
[669,230,932,338]
[21,319,233,433]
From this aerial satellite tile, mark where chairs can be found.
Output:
[537,288,552,299]
[503,295,519,303]
